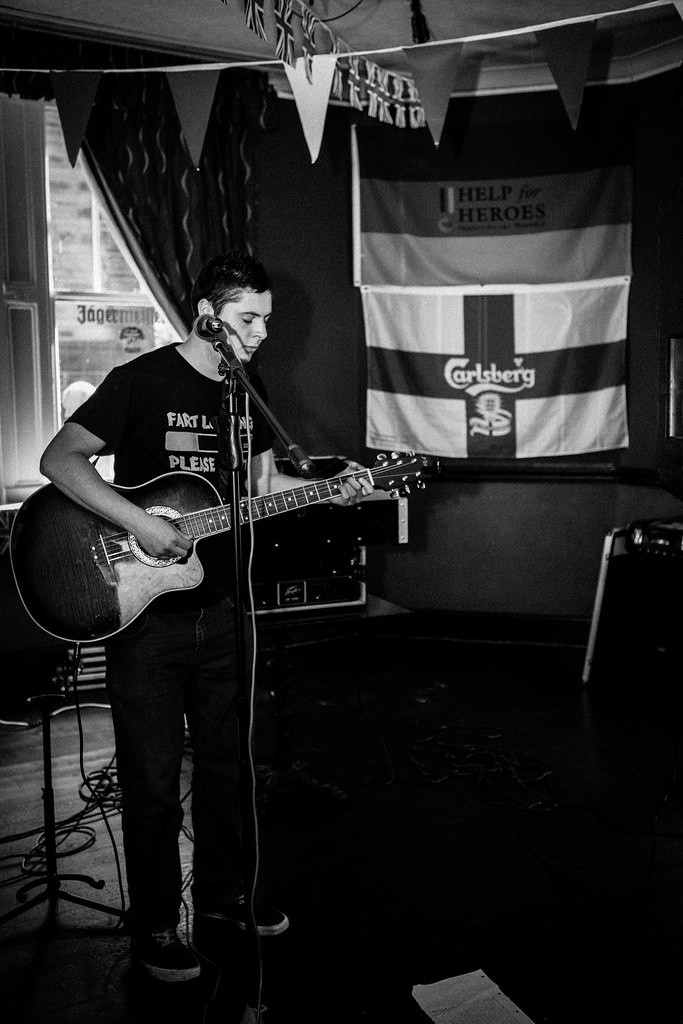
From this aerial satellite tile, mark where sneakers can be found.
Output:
[129,928,200,982]
[193,894,289,935]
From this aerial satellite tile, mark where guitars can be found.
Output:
[10,448,440,644]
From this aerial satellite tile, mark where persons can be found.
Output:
[39,249,375,983]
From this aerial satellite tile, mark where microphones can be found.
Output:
[194,313,224,340]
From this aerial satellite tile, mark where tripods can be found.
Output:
[0,694,125,926]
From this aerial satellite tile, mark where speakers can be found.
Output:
[580,517,683,708]
